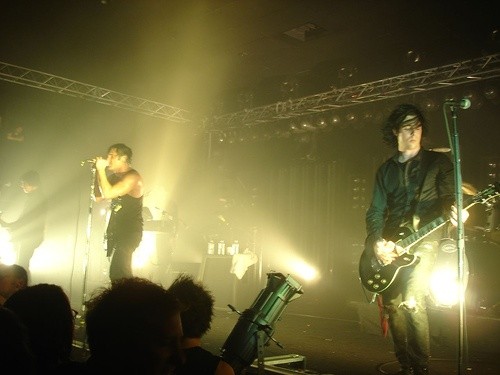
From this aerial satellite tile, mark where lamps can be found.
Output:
[288,81,500,135]
[406,50,421,67]
[218,270,304,375]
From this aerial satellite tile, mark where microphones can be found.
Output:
[85,158,108,165]
[444,98,472,110]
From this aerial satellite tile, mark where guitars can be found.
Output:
[358,181,500,295]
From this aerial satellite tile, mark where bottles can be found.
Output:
[208,240,215,255]
[218,240,225,256]
[231,240,240,255]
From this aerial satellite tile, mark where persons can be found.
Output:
[169,273,235,375]
[0,171,49,268]
[365,104,470,375]
[89,143,145,286]
[0,263,78,375]
[82,277,186,375]
